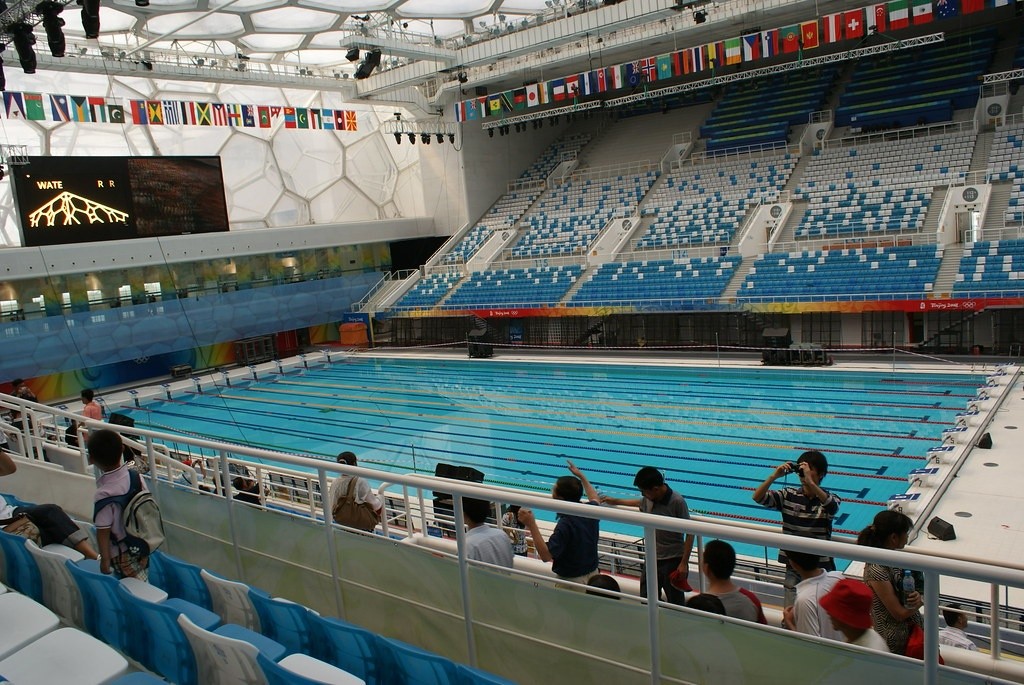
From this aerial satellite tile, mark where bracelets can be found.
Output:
[97,553,101,560]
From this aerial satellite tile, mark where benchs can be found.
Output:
[699,25,1024,159]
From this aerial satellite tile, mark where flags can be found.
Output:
[454,48,688,125]
[4,91,124,124]
[130,98,357,132]
[687,0,1023,72]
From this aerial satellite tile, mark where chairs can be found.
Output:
[388,130,1024,305]
[0,487,513,685]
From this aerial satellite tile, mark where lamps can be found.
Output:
[458,65,468,83]
[36,0,68,57]
[693,9,708,24]
[0,43,6,91]
[392,132,455,144]
[487,47,922,139]
[9,21,39,74]
[76,0,101,39]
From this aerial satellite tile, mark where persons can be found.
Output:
[88,430,150,586]
[519,459,600,595]
[683,540,768,626]
[751,451,841,609]
[463,496,514,576]
[600,466,696,609]
[0,446,102,560]
[502,504,528,557]
[65,388,103,453]
[198,475,271,512]
[782,511,979,667]
[0,383,51,476]
[329,451,383,538]
[584,574,622,603]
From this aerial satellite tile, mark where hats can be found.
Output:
[818,578,875,629]
[669,570,693,592]
[182,459,193,466]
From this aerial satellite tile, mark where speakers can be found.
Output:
[978,433,992,449]
[927,516,956,541]
[468,329,493,355]
[761,328,793,362]
[346,49,360,62]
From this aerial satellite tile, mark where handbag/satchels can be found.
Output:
[905,623,945,666]
[333,475,384,530]
[3,512,43,550]
[127,445,151,474]
[503,512,520,546]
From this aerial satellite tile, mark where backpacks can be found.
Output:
[94,468,167,559]
